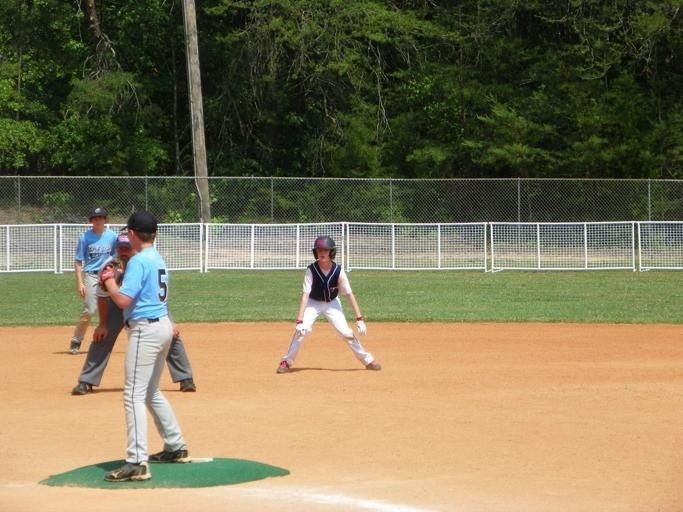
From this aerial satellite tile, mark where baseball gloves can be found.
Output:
[98,258,123,291]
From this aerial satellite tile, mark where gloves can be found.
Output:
[295,320,304,336]
[356,316,366,336]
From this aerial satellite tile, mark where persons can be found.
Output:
[99,211,192,483]
[276,236,382,375]
[69,233,197,394]
[68,205,119,354]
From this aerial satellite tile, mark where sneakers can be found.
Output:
[71,382,92,395]
[69,340,80,353]
[276,361,288,373]
[366,361,381,370]
[148,446,191,463]
[104,462,151,483]
[179,378,196,392]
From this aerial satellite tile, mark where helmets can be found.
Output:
[311,236,335,260]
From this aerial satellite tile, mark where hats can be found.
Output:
[116,233,131,250]
[119,210,157,232]
[89,207,107,218]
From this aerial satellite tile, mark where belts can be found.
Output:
[86,271,97,274]
[125,318,159,327]
